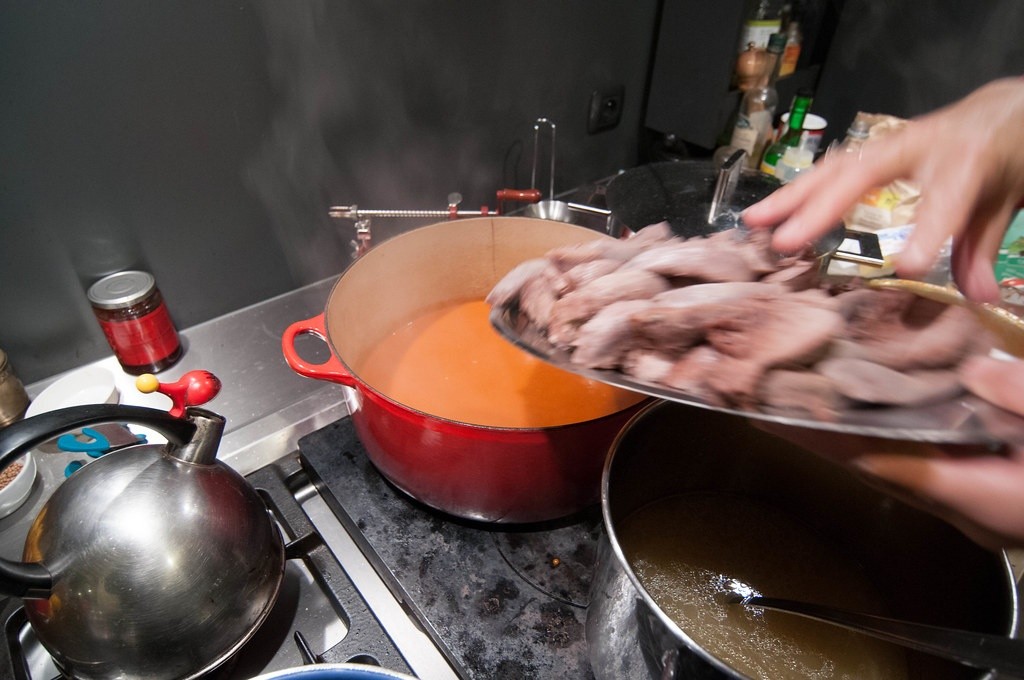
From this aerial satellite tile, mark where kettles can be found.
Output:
[0,371,289,680]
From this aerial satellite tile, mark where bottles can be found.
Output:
[733,0,805,85]
[726,33,788,172]
[775,148,817,186]
[757,93,812,176]
[832,118,870,150]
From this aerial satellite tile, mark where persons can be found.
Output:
[741,73,1024,554]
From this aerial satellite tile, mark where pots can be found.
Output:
[566,149,885,279]
[284,216,660,527]
[584,397,1024,680]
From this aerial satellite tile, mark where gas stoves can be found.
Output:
[0,462,421,680]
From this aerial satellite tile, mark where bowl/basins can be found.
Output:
[25,369,120,436]
[0,451,39,519]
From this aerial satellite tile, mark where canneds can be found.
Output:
[88,270,181,375]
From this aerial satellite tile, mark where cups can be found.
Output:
[778,110,829,159]
